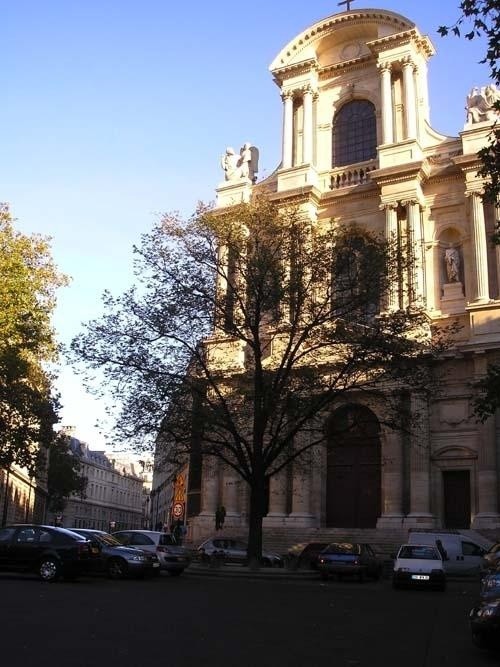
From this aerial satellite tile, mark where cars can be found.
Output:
[197,536,283,568]
[0,523,192,584]
[389,525,500,656]
[316,541,381,583]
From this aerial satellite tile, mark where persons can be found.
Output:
[145,504,227,569]
[220,147,241,180]
[234,143,252,178]
[435,538,449,589]
[466,85,500,124]
[444,242,460,283]
